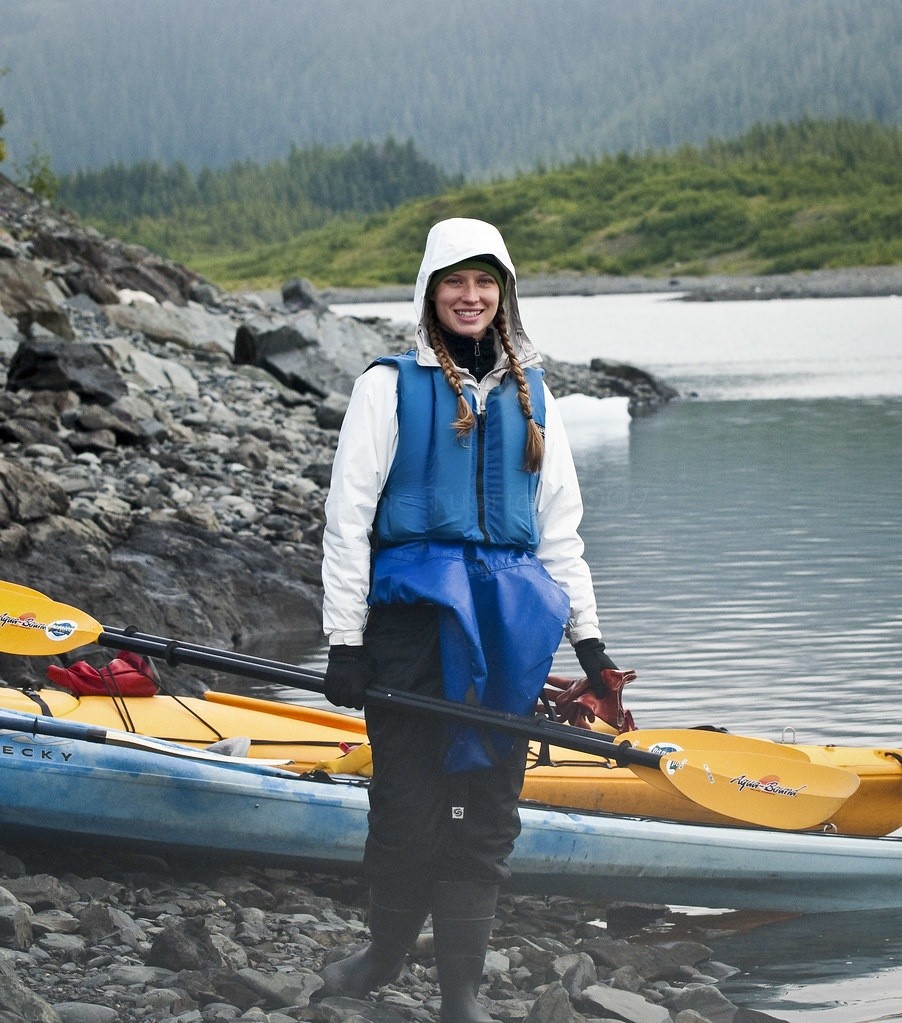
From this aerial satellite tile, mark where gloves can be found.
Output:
[534,668,638,731]
[575,638,620,700]
[322,645,380,711]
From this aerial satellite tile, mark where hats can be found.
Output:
[424,253,508,308]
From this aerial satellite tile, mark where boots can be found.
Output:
[316,885,433,996]
[433,880,502,1023]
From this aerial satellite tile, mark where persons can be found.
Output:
[323,218,620,1023]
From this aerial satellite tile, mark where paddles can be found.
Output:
[0,580,811,803]
[0,590,861,830]
[0,716,296,766]
[194,690,366,736]
